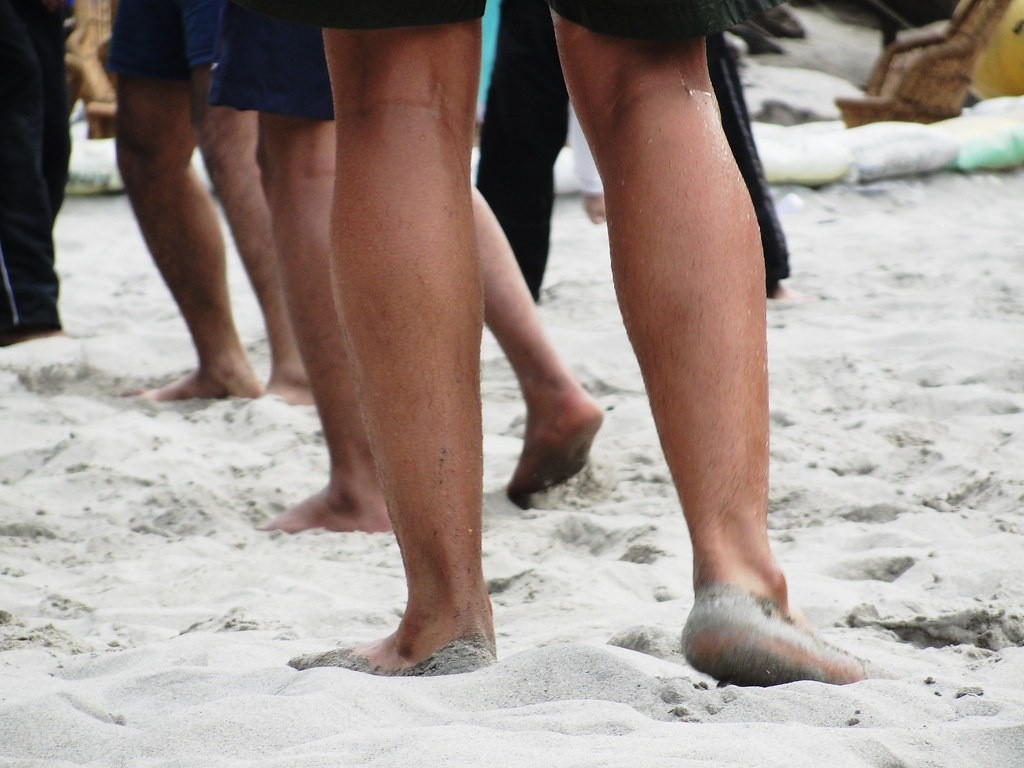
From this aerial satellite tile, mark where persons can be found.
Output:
[0,0,865,689]
